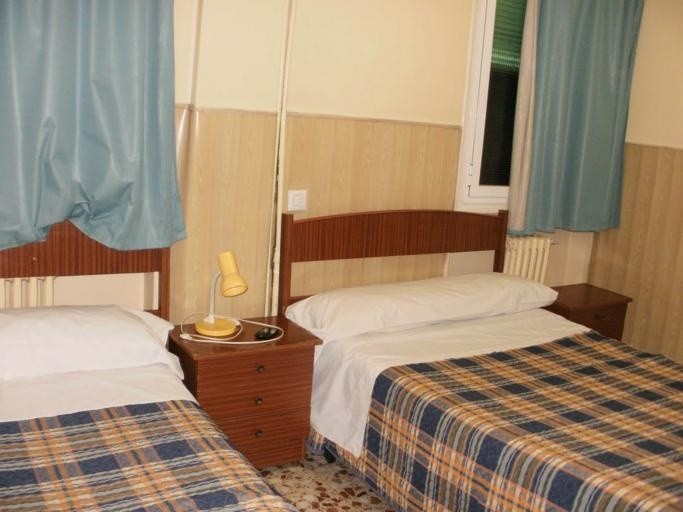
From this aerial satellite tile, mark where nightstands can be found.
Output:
[167,315,322,470]
[542,283,633,342]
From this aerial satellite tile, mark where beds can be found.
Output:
[2,222,305,512]
[280,210,683,512]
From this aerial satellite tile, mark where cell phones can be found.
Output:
[255,327,279,339]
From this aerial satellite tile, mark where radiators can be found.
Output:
[502,236,551,283]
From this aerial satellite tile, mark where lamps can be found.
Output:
[194,250,247,338]
